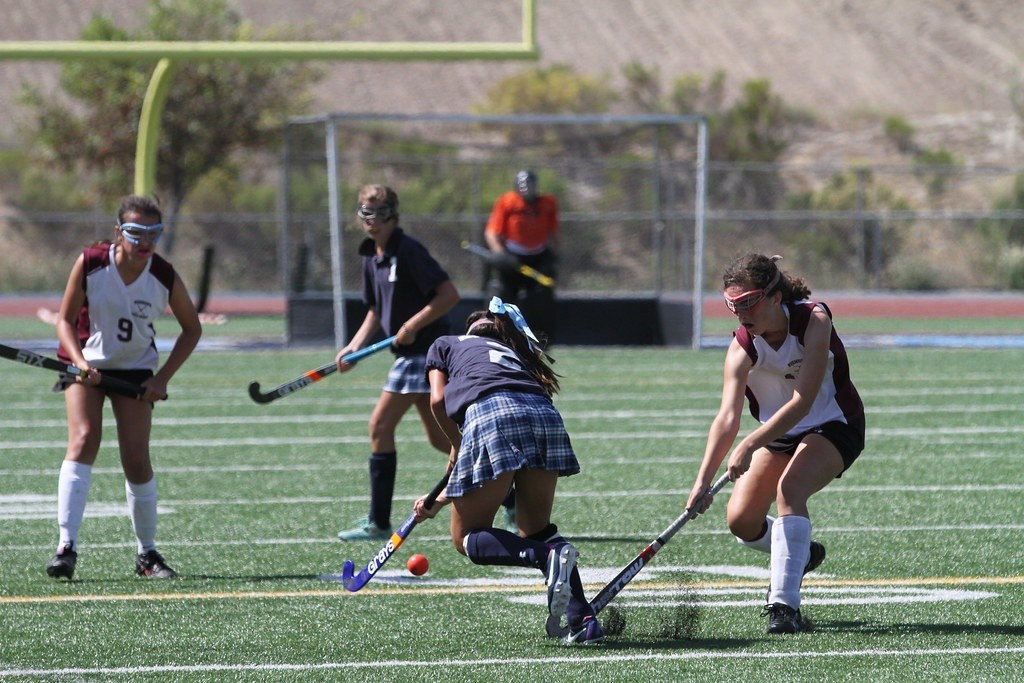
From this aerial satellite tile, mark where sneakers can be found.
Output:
[47,539,77,580]
[338,515,392,541]
[502,508,520,535]
[542,542,579,618]
[767,540,825,604]
[760,602,801,635]
[561,615,605,645]
[135,550,178,579]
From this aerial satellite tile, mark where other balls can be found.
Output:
[407,554,428,576]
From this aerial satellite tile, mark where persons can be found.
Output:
[484,168,558,348]
[685,253,866,634]
[47,196,203,579]
[424,296,604,643]
[335,185,521,541]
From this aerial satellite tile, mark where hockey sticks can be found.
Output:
[461,240,557,287]
[0,344,168,401]
[343,467,451,593]
[248,334,397,405]
[546,469,730,639]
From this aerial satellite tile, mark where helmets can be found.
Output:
[516,170,536,203]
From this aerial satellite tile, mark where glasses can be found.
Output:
[117,218,163,246]
[357,205,392,220]
[723,288,767,318]
